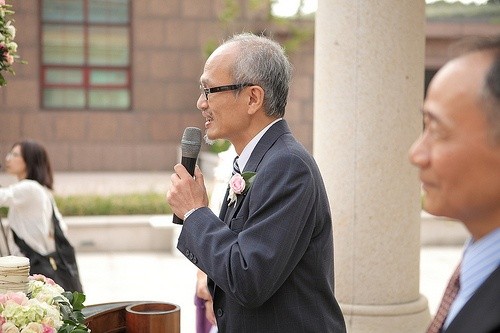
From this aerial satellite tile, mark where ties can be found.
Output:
[425,261,460,333]
[232,156,241,176]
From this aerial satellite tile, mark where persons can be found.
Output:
[166,32,347,333]
[0,141,83,293]
[408,33,500,333]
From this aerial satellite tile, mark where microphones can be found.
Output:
[172,126,202,224]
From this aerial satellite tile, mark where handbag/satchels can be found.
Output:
[10,247,83,293]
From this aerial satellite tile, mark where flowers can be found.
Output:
[226,172,256,209]
[0,274,91,333]
[0,0,28,87]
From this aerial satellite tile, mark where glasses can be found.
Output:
[7,150,23,159]
[199,83,254,101]
